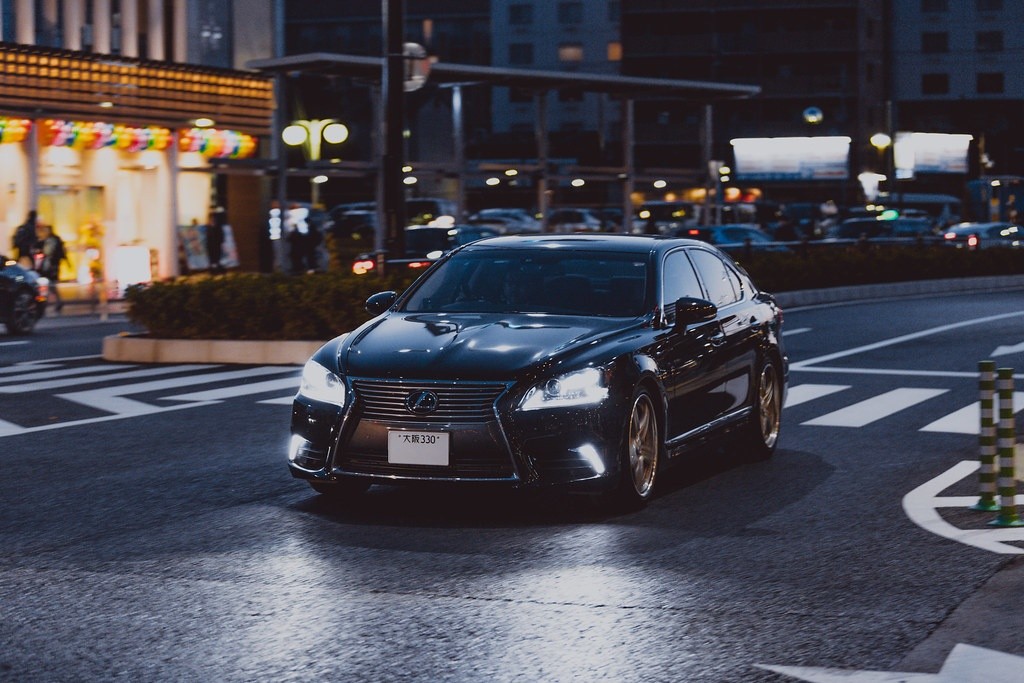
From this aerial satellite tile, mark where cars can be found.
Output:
[287,196,1023,274]
[287,232,789,512]
[0,255,50,335]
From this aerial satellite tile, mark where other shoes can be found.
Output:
[53,302,64,312]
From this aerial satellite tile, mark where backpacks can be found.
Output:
[11,225,28,248]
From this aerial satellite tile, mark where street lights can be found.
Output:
[281,115,348,200]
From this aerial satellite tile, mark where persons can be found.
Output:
[12,210,71,309]
[455,266,539,314]
[204,213,225,271]
[291,218,328,274]
